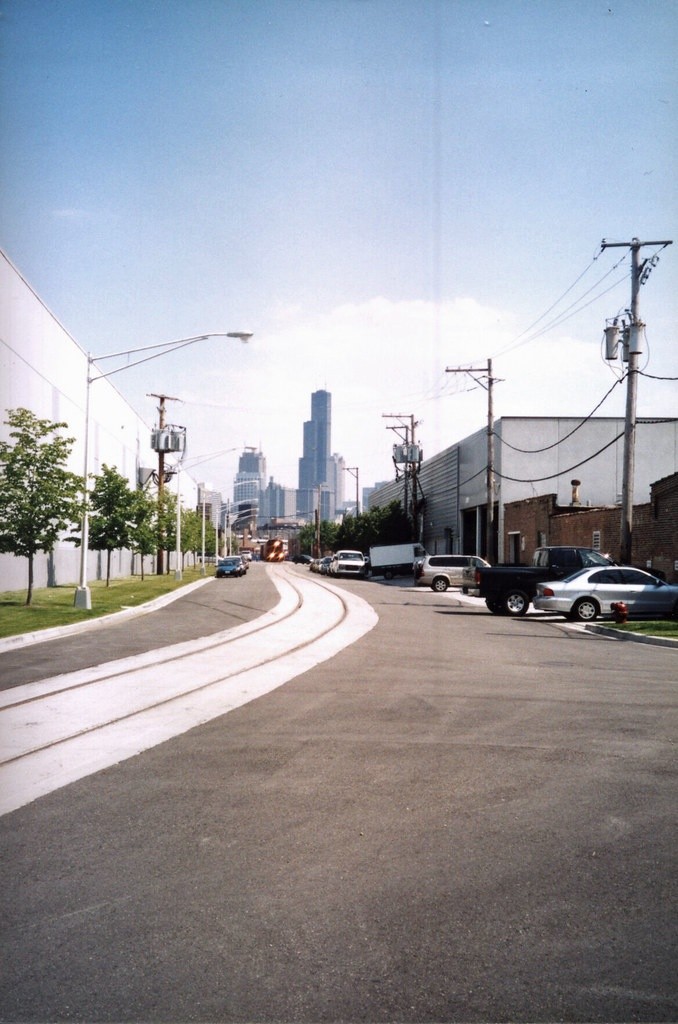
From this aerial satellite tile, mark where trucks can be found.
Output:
[369,543,431,580]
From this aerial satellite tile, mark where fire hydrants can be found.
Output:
[610,602,630,624]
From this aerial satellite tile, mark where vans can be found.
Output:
[414,554,492,592]
[334,549,367,579]
[242,550,253,561]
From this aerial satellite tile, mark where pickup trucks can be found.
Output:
[462,546,667,617]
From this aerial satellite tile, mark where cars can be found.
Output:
[292,555,314,564]
[310,554,336,575]
[532,566,678,621]
[215,555,249,578]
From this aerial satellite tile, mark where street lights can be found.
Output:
[174,446,258,583]
[201,479,259,576]
[73,332,256,609]
[224,507,259,557]
[216,498,258,565]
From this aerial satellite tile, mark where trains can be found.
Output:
[264,539,285,562]
